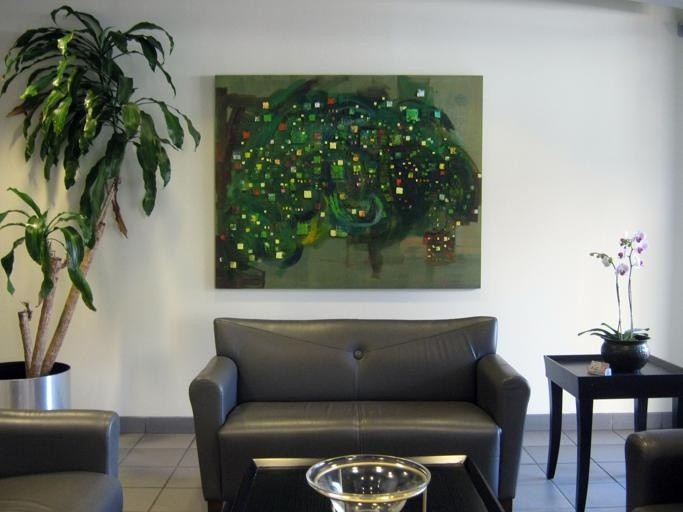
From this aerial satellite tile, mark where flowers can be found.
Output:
[577,232,650,343]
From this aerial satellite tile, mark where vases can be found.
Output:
[602,333,652,375]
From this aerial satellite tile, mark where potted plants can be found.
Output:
[1,1,202,410]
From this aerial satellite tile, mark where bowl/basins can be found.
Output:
[305,452,432,512]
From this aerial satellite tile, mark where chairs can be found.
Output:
[1,411,125,512]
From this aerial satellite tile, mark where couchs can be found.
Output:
[188,314,533,512]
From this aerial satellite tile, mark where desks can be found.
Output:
[543,351,683,512]
